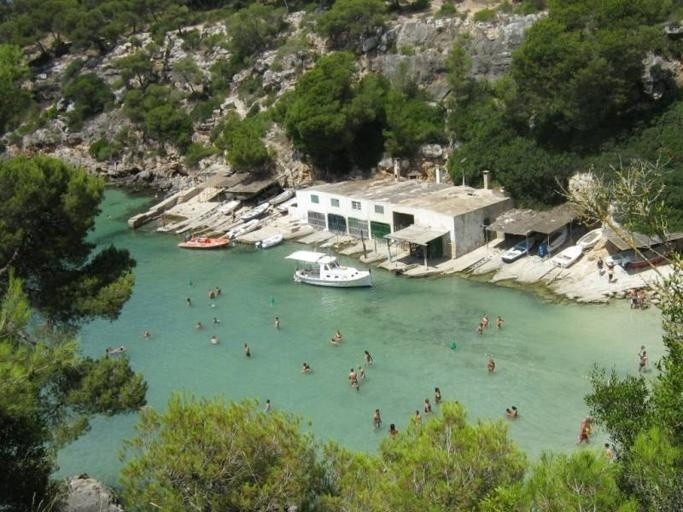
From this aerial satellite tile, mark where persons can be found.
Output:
[348,351,373,390]
[330,330,344,345]
[636,287,647,310]
[244,342,253,357]
[638,348,648,374]
[303,362,312,374]
[627,286,638,309]
[183,286,223,344]
[605,261,615,284]
[476,315,503,335]
[274,317,281,329]
[580,416,594,435]
[506,405,517,418]
[266,400,271,411]
[487,360,495,375]
[577,429,590,444]
[596,255,604,276]
[372,388,441,436]
[602,443,614,461]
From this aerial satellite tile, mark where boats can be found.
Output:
[254,233,283,251]
[501,225,678,269]
[176,234,229,249]
[216,188,298,240]
[285,250,373,288]
